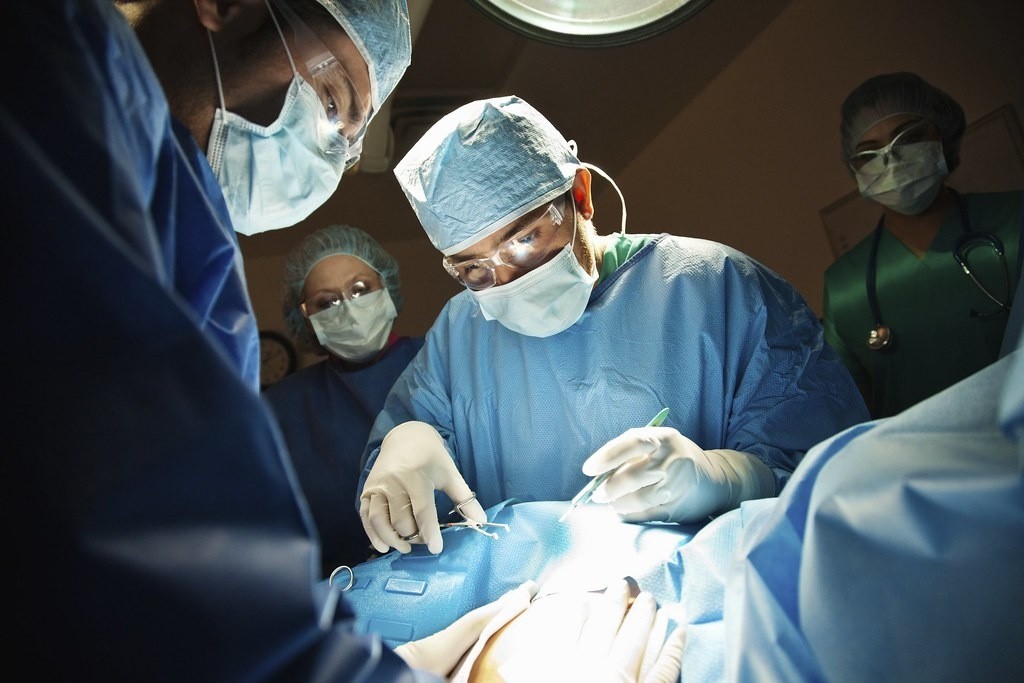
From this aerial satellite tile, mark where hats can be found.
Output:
[393,96,587,257]
[318,0,412,116]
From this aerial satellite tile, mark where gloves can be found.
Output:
[360,421,488,554]
[582,427,775,523]
[393,579,686,683]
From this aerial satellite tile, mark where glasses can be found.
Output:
[441,193,566,293]
[271,4,369,174]
[845,118,942,171]
[299,277,384,322]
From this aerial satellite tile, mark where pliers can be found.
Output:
[397,491,510,542]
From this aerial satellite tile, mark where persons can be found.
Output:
[261,71,871,558]
[1,0,690,683]
[812,76,1022,422]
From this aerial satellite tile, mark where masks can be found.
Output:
[855,141,947,216]
[462,189,600,338]
[308,287,398,362]
[204,1,351,238]
